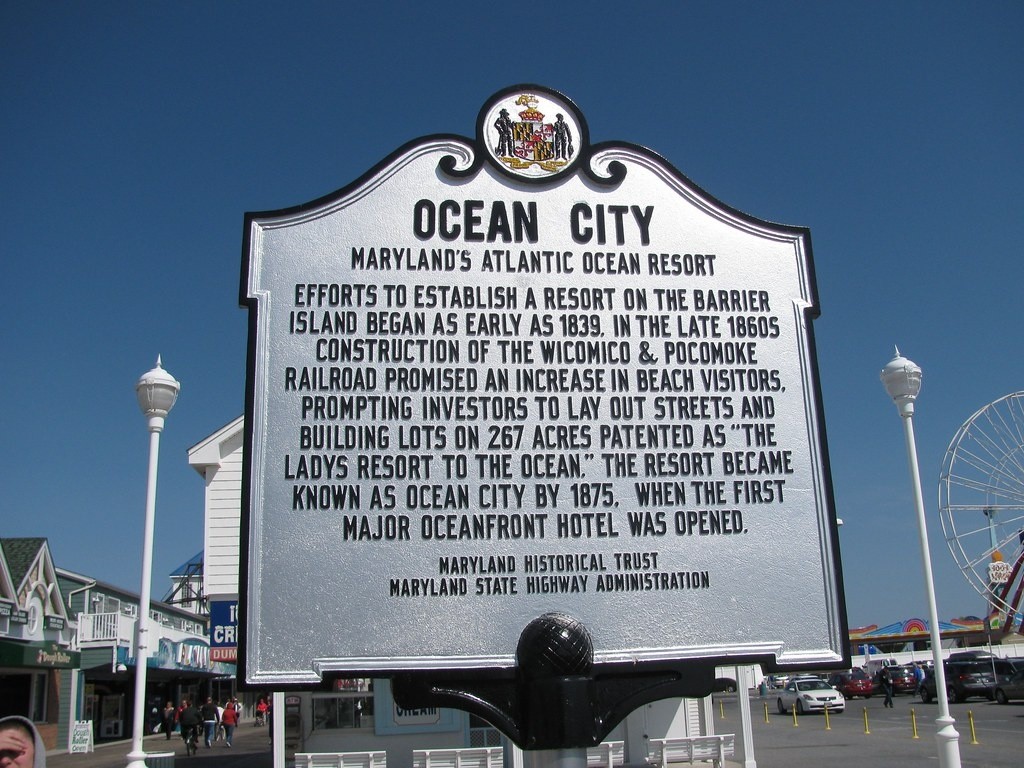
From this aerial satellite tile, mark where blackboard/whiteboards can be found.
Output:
[70,720,94,753]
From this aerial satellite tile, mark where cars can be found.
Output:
[777,677,845,715]
[771,649,1024,706]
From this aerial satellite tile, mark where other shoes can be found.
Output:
[890,704,893,708]
[208,740,211,747]
[227,742,231,747]
[883,702,889,708]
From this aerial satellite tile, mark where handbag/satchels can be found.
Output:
[888,679,892,684]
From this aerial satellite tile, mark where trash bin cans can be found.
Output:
[145,751,177,768]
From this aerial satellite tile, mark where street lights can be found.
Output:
[880,343,963,767]
[123,351,185,768]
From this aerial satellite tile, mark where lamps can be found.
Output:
[92,597,100,604]
[115,663,128,672]
[163,617,169,623]
[125,607,132,613]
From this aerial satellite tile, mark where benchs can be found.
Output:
[294,750,387,768]
[413,746,503,768]
[643,734,736,768]
[587,741,625,768]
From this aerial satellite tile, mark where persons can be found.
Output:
[163,696,273,754]
[0,716,47,768]
[913,664,926,699]
[879,665,894,708]
